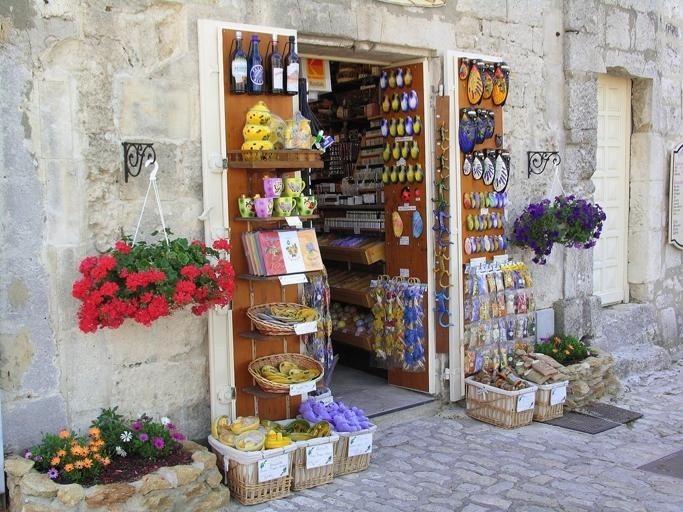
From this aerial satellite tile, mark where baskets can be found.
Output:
[273,419,339,491]
[247,353,324,394]
[464,375,537,430]
[206,433,298,506]
[246,302,322,336]
[532,380,568,422]
[296,412,377,479]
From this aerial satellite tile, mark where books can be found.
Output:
[241,227,324,277]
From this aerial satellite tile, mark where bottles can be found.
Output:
[229,29,300,96]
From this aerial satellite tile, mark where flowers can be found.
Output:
[70,226,235,334]
[512,194,606,264]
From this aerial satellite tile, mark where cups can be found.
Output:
[237,177,317,219]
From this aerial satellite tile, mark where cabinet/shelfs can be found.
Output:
[314,227,384,352]
[226,149,326,398]
